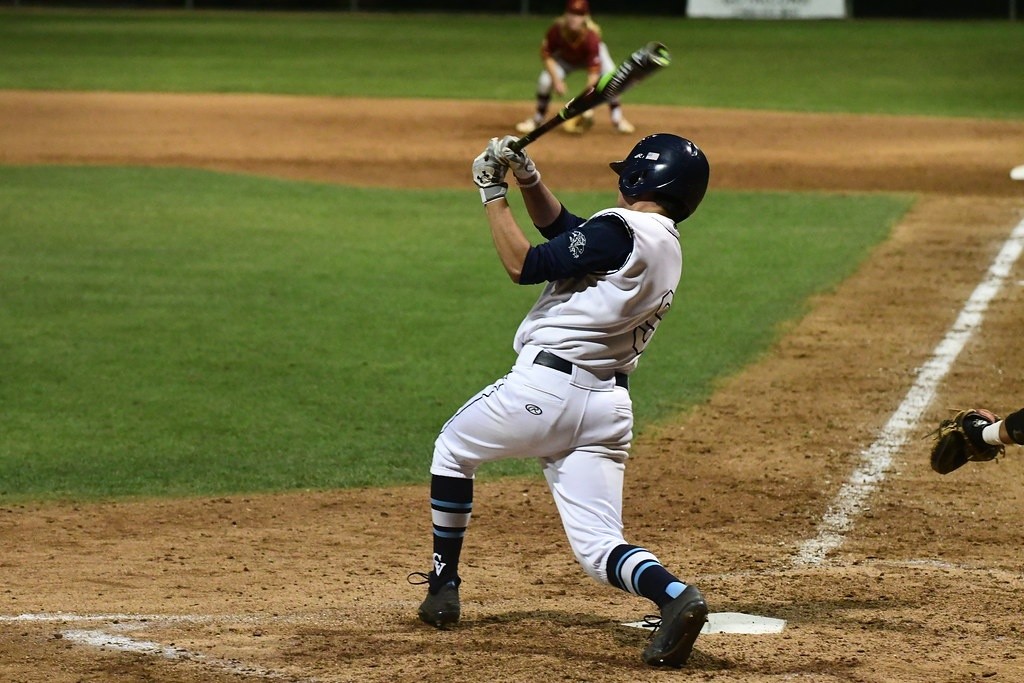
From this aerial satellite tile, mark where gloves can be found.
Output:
[472,137,509,204]
[495,135,541,188]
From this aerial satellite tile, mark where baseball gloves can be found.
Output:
[929,407,1005,475]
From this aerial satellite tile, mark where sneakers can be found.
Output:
[642,585,709,668]
[407,570,461,628]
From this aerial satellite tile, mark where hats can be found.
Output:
[567,0,588,15]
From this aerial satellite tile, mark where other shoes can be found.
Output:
[612,114,634,133]
[516,118,539,133]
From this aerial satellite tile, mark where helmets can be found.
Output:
[609,133,710,224]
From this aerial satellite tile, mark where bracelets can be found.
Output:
[982,420,1005,446]
[582,109,593,118]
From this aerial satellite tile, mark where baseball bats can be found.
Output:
[510,42,671,150]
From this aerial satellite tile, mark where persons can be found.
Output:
[407,133,710,667]
[516,0,637,135]
[930,408,1024,475]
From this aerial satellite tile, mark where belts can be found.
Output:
[533,351,628,389]
